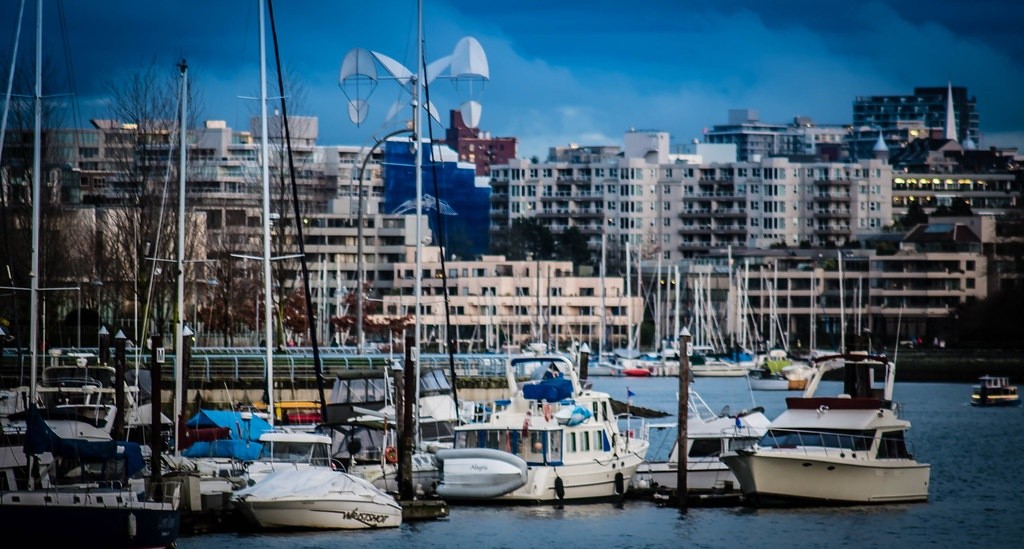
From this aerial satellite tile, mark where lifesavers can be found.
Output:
[545,407,550,422]
[386,447,398,463]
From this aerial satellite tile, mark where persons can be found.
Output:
[346,336,358,346]
[259,337,266,353]
[288,339,295,347]
[912,335,923,349]
[331,337,338,347]
[933,336,945,349]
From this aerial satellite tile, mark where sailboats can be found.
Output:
[0,1,826,549]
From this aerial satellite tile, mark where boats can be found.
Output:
[719,351,933,507]
[970,374,1021,407]
